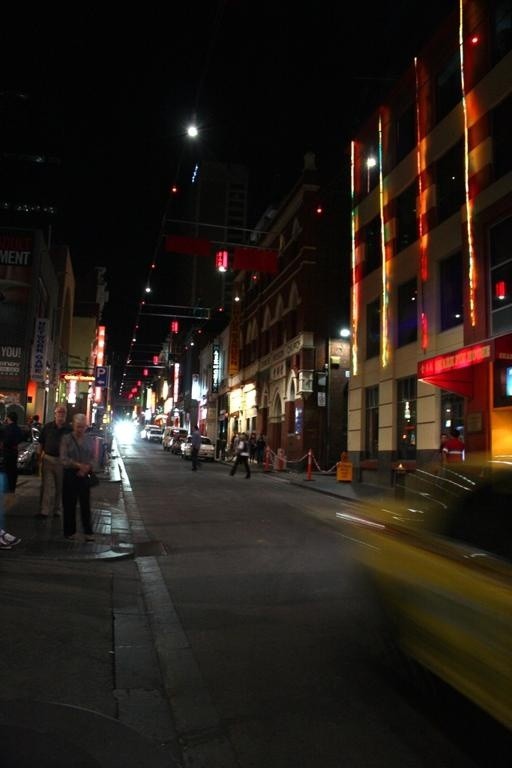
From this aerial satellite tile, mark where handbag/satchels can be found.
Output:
[80,471,99,489]
[238,441,249,453]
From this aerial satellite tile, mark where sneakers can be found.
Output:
[0,529,23,550]
[85,533,96,541]
[65,533,78,540]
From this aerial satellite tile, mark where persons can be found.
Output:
[437,432,449,456]
[190,424,204,472]
[215,430,267,468]
[442,428,465,465]
[0,401,113,549]
[226,434,251,479]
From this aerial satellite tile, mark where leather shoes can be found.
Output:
[191,462,202,472]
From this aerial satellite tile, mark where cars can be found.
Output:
[16,426,40,474]
[140,425,216,462]
[335,455,512,731]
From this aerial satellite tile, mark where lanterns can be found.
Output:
[127,355,160,400]
[496,280,506,302]
[215,249,229,274]
[170,319,179,333]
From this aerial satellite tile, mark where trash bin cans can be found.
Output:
[86,435,104,466]
